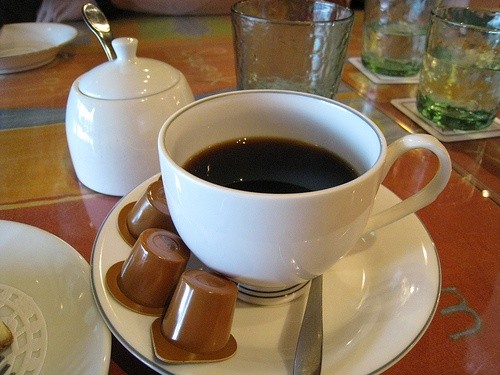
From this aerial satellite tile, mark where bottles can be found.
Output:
[63,34,198,201]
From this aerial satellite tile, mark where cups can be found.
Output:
[231,0,355,100]
[360,0,444,78]
[416,5,500,132]
[156,87,453,308]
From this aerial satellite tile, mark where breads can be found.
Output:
[0,320,14,353]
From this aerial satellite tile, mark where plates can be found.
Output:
[0,22,78,76]
[0,219,112,374]
[89,171,443,375]
